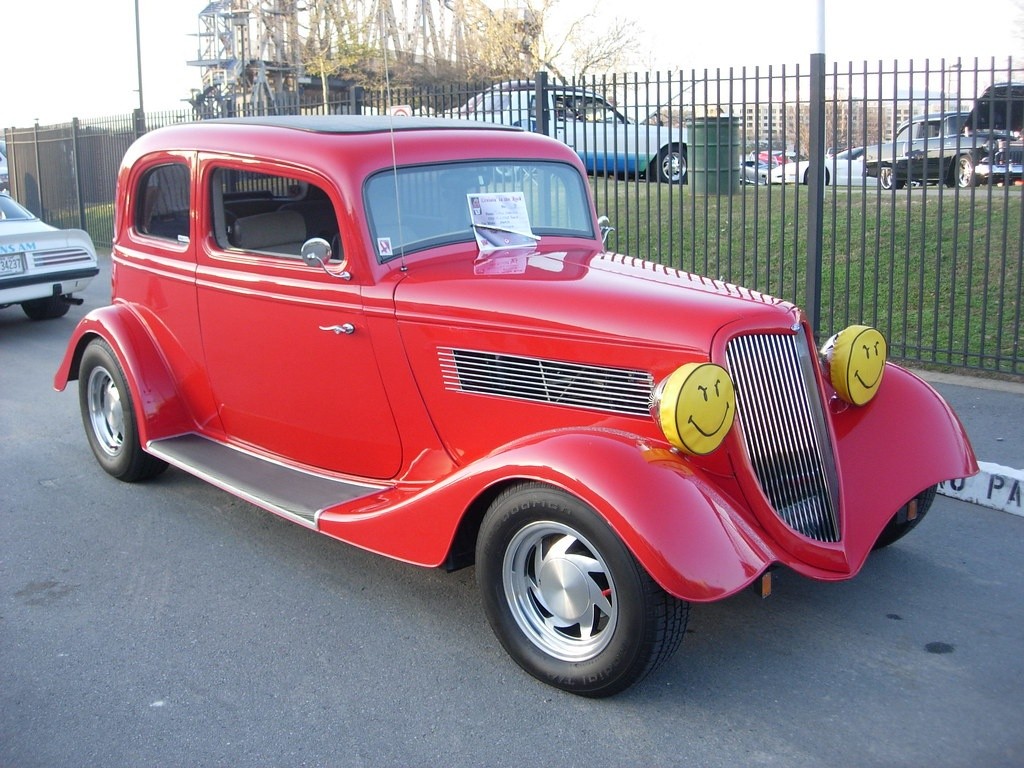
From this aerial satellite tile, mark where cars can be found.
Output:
[0,140,10,192]
[51,114,981,700]
[1,192,100,321]
[433,80,1023,190]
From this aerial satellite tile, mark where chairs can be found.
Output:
[223,195,418,259]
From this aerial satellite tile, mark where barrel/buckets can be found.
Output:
[684,115,740,197]
